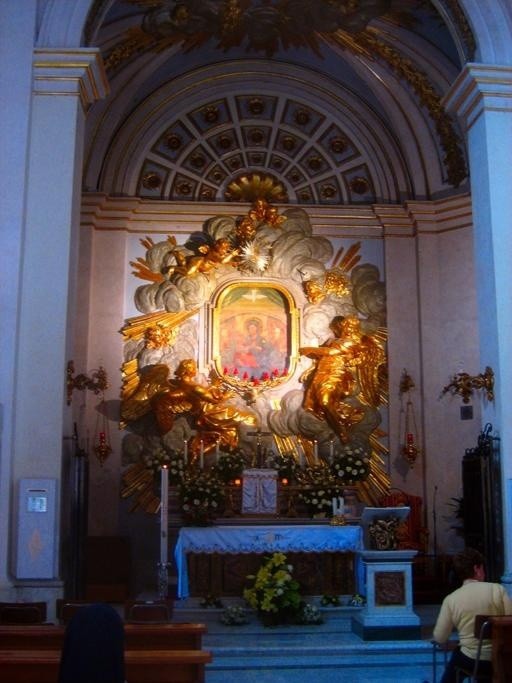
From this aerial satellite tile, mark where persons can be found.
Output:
[237,318,269,374]
[433,547,512,683]
[298,316,360,444]
[167,238,240,275]
[175,359,257,465]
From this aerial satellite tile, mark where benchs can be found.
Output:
[0,597,216,681]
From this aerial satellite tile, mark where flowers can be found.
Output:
[263,443,373,522]
[149,442,247,527]
[236,550,304,614]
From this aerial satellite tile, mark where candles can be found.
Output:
[328,439,334,462]
[405,400,414,436]
[159,462,170,566]
[200,438,204,469]
[183,439,188,466]
[312,439,318,463]
[297,438,304,468]
[215,442,220,465]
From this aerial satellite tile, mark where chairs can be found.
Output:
[428,606,511,682]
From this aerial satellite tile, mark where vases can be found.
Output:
[253,602,288,628]
